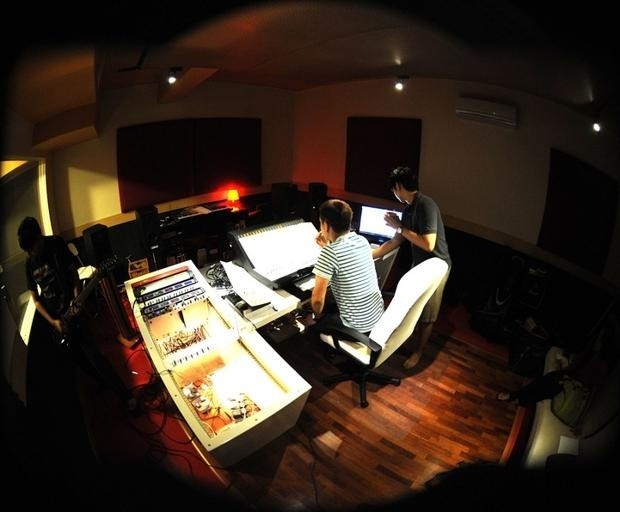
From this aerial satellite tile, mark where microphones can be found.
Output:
[68,242,79,256]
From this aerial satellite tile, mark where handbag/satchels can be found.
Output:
[552,375,595,432]
[468,285,510,342]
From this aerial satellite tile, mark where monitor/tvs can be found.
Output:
[356,204,405,245]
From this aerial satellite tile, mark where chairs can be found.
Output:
[319,256,450,409]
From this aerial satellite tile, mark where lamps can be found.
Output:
[227,189,240,213]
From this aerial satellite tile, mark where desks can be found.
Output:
[199,243,400,329]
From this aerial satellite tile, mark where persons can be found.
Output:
[17,216,138,411]
[497,235,620,406]
[306,200,385,377]
[371,165,452,369]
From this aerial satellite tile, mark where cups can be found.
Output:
[387,212,397,219]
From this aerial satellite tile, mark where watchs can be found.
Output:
[396,224,404,235]
[311,311,320,320]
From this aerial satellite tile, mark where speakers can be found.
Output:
[135,205,158,231]
[271,182,298,217]
[308,182,328,213]
[82,224,114,265]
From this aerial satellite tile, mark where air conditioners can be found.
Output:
[454,97,517,129]
